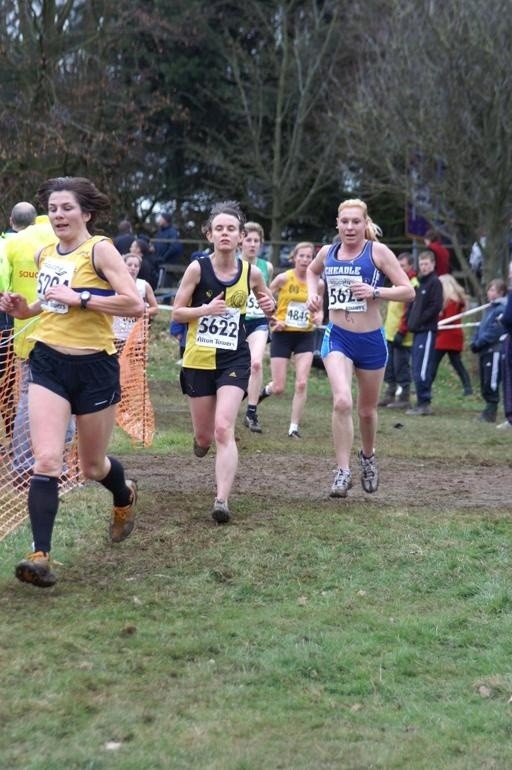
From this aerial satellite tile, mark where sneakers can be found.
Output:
[328,471,353,496]
[14,553,57,588]
[193,438,208,457]
[375,400,394,409]
[404,404,432,415]
[109,480,139,541]
[287,431,300,441]
[211,500,230,524]
[357,450,379,494]
[243,416,262,433]
[385,402,410,410]
[496,423,509,430]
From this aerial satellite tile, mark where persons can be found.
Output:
[171,200,278,524]
[0,200,37,444]
[279,236,297,267]
[257,241,326,440]
[150,212,182,264]
[470,277,508,423]
[112,220,137,255]
[170,251,209,359]
[305,198,416,498]
[128,237,159,293]
[0,176,145,588]
[406,250,444,415]
[423,230,450,276]
[497,259,512,430]
[1,181,78,490]
[432,274,473,398]
[114,253,160,423]
[379,252,420,410]
[236,222,275,433]
[469,239,481,281]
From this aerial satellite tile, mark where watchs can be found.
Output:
[372,287,379,301]
[79,291,91,310]
[272,299,278,313]
